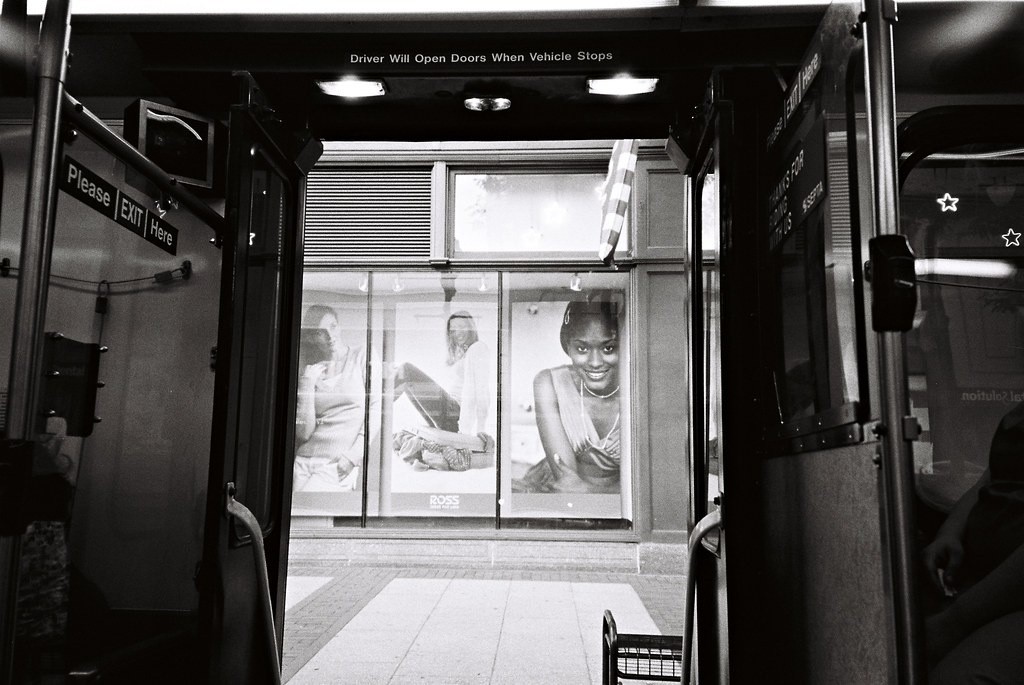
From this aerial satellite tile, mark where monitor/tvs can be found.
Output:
[123,99,214,189]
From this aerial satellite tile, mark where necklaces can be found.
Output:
[578,380,620,448]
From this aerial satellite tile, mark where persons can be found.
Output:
[394,311,491,436]
[918,399,1024,685]
[290,306,368,491]
[511,301,621,494]
